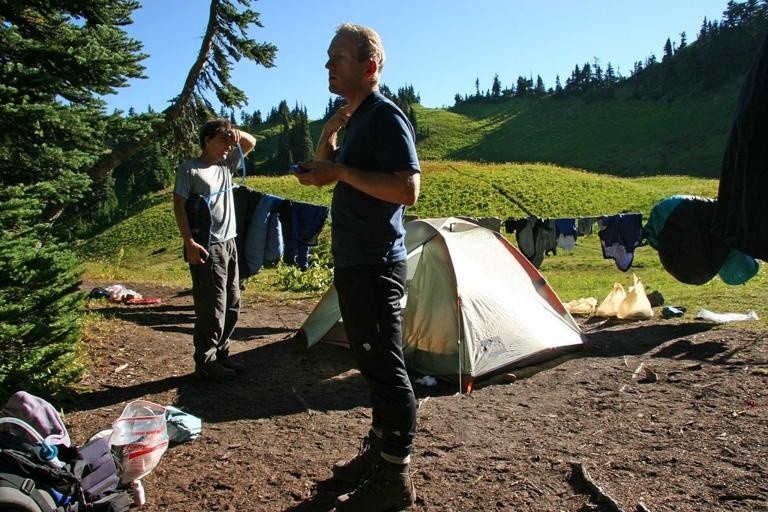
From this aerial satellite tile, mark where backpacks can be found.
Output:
[88,286,112,300]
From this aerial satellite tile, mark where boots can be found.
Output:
[337,461,417,511]
[335,437,389,482]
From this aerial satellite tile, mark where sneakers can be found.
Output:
[193,361,234,383]
[217,354,245,378]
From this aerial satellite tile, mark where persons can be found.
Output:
[173,120,258,383]
[287,21,422,508]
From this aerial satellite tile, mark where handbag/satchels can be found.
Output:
[162,403,203,444]
[1,388,71,450]
[0,433,91,512]
[107,397,170,488]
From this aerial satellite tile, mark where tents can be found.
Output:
[289,214,593,390]
